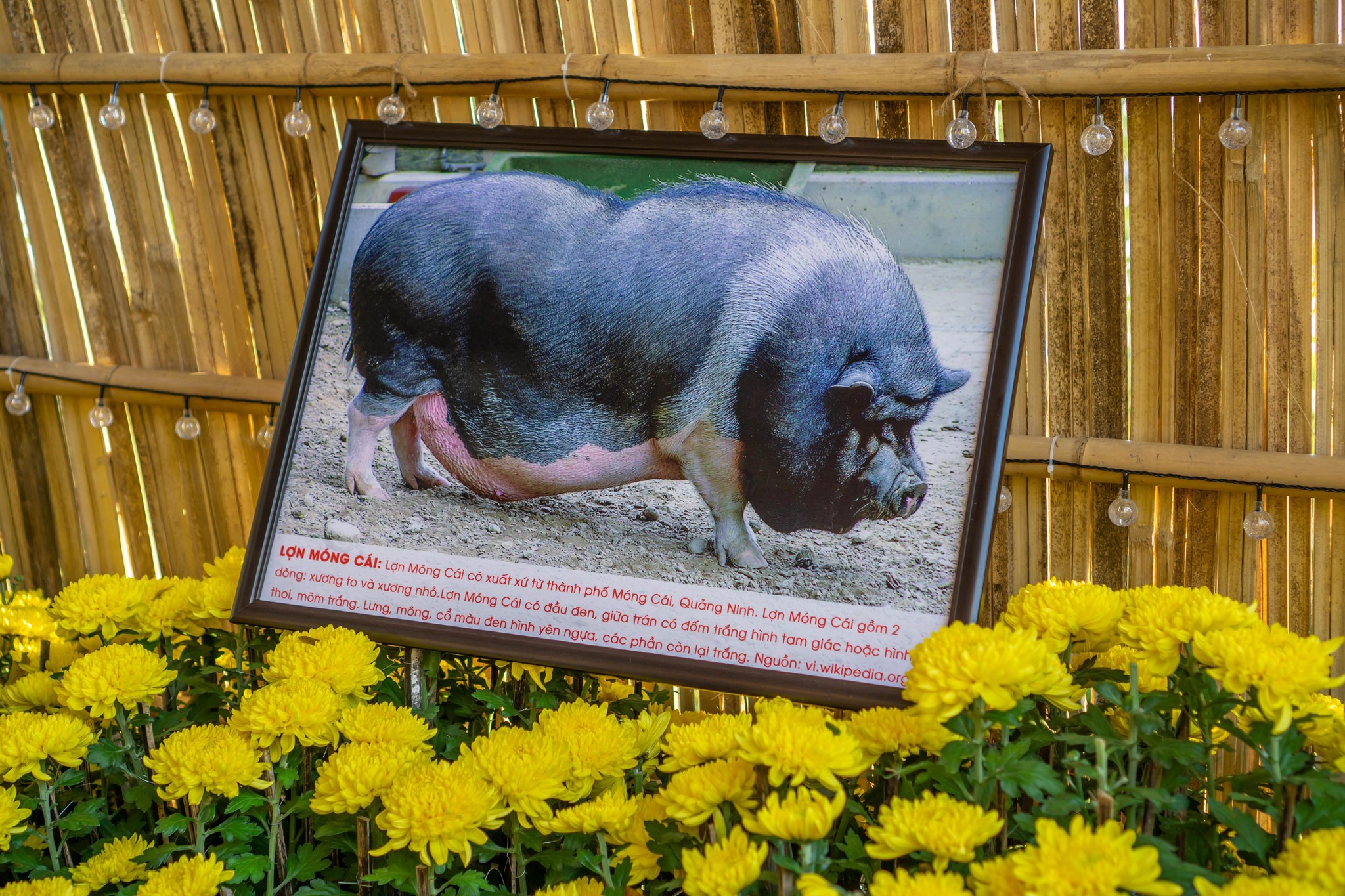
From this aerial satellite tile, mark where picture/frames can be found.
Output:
[230,118,1054,712]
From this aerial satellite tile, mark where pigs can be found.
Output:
[333,166,976,573]
